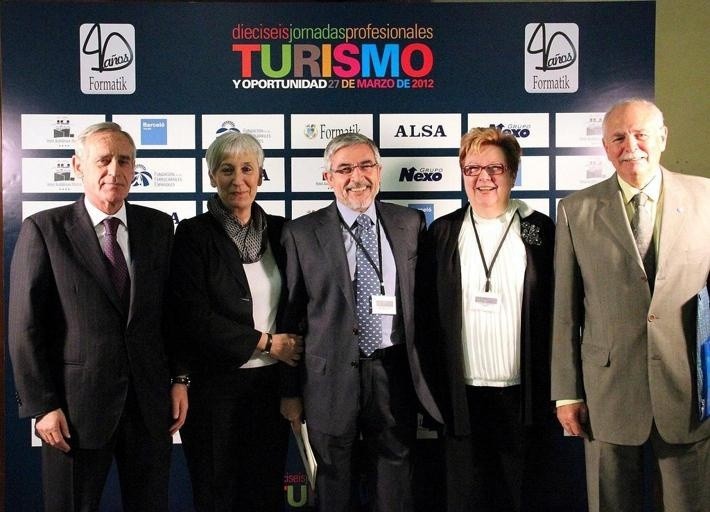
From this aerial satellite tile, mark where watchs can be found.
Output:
[261,332,273,355]
[169,376,192,387]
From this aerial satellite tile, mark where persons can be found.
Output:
[550,96,710,512]
[8,123,196,511]
[168,129,317,511]
[414,126,587,512]
[277,132,446,512]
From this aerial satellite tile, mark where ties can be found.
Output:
[355,214,384,359]
[629,192,656,299]
[102,218,128,300]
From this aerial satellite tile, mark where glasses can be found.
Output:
[331,164,380,175]
[463,164,504,177]
